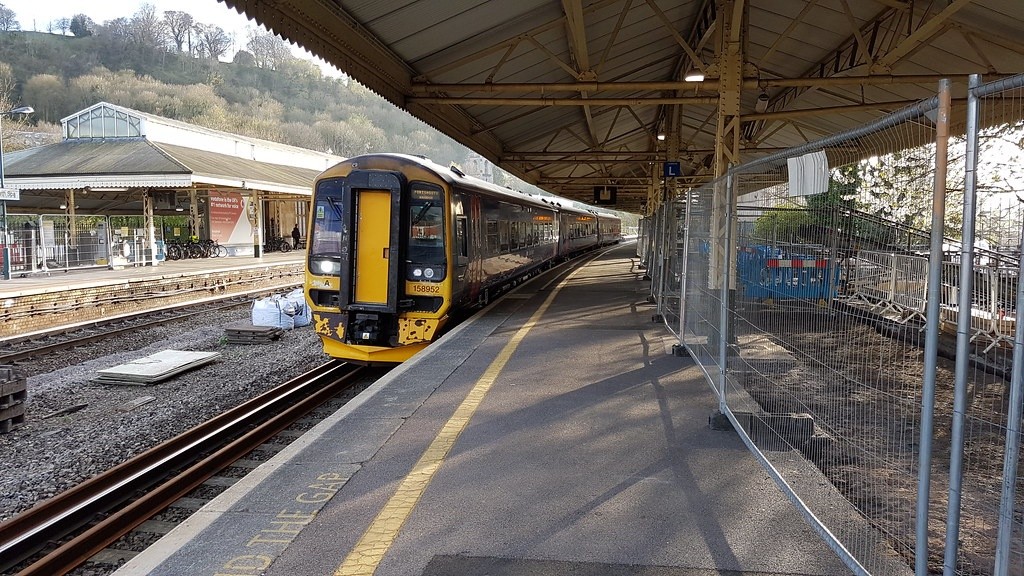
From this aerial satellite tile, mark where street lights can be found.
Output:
[0,106,35,281]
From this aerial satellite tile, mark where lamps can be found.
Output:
[60,198,66,209]
[176,201,184,212]
[657,128,665,140]
[755,93,770,113]
[685,68,704,81]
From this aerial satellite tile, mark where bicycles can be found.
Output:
[165,238,228,260]
[263,235,291,253]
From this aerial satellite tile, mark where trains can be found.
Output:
[303,152,621,367]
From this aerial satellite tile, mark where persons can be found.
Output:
[292,223,300,249]
